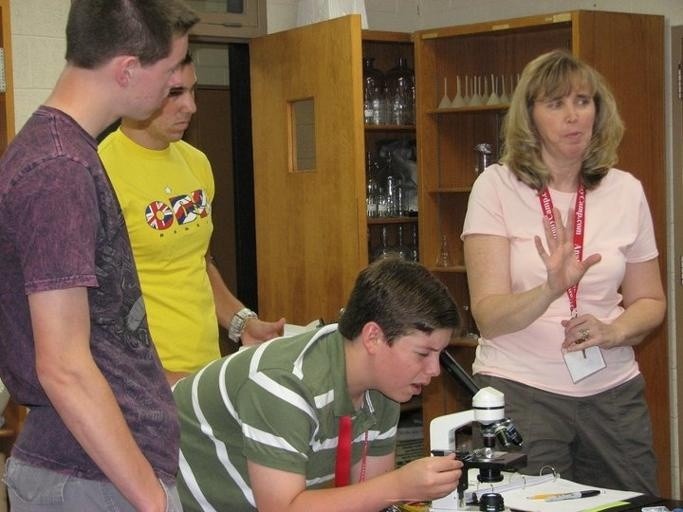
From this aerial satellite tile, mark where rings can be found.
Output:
[579,328,591,341]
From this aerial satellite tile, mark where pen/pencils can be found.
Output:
[526,490,601,501]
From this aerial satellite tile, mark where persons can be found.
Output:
[88,47,288,388]
[165,250,465,511]
[457,47,668,502]
[0,1,204,512]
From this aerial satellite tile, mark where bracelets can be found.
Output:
[225,307,257,344]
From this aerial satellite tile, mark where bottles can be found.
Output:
[364,55,417,263]
[432,233,454,266]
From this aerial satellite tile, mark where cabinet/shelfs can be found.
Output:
[0,0,27,458]
[361,9,671,500]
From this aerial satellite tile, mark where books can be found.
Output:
[454,466,646,511]
